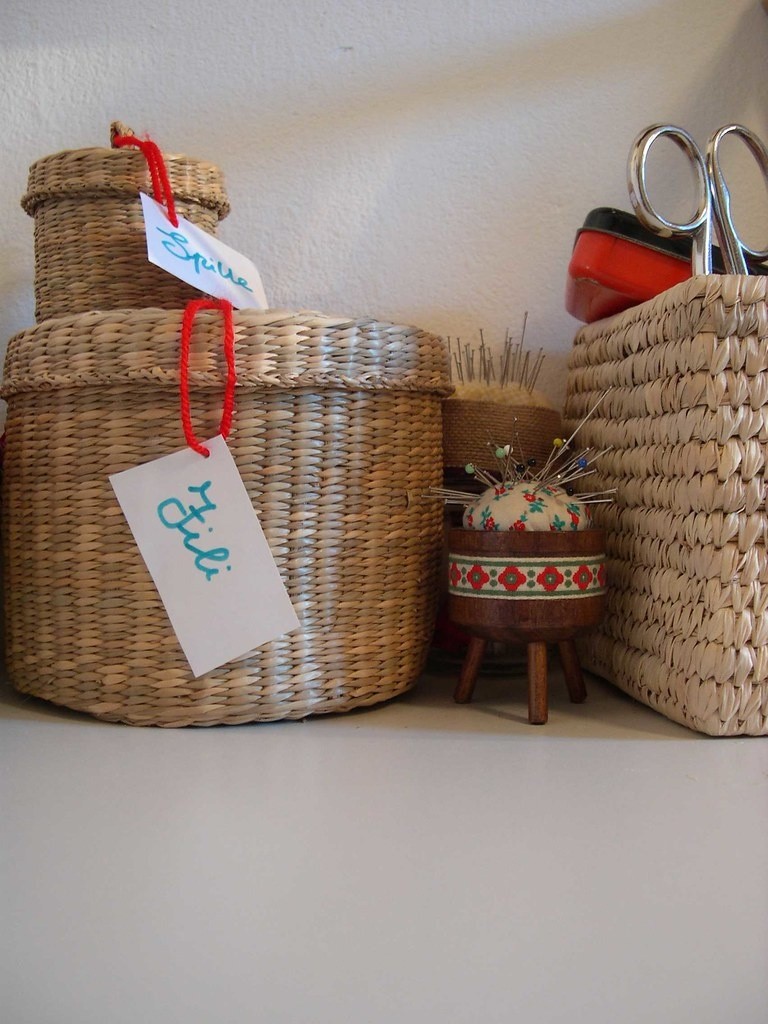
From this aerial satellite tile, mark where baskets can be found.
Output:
[0,284,455,726]
[560,274,768,739]
[22,120,232,324]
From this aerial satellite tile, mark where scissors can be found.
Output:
[626,121,768,275]
